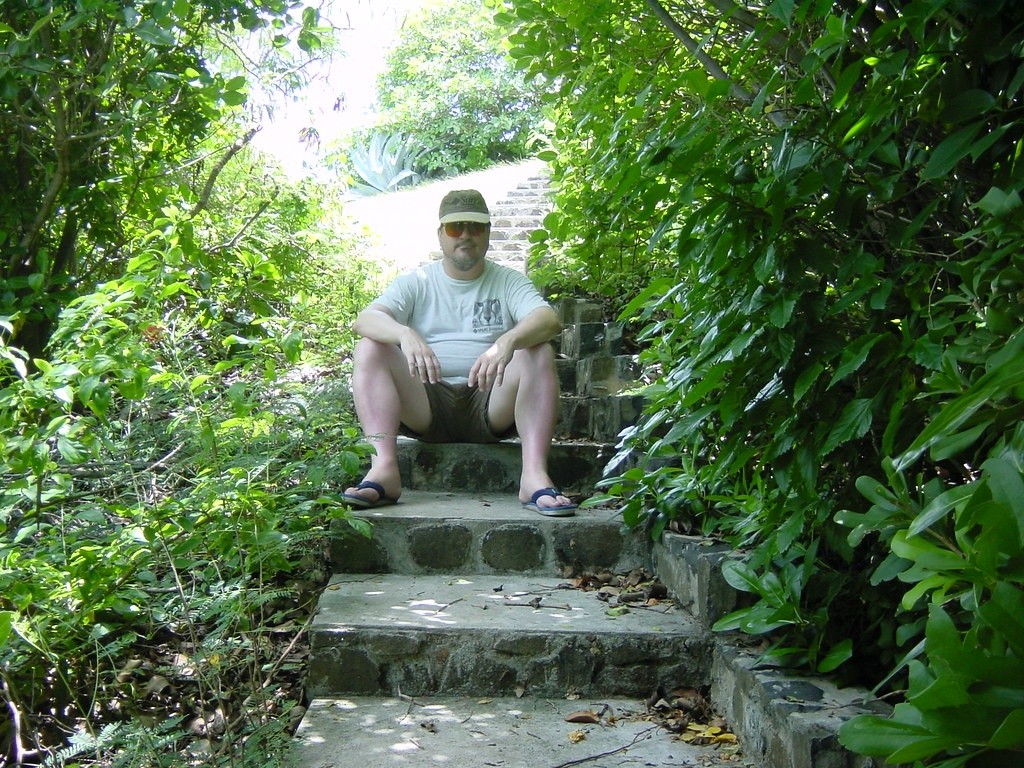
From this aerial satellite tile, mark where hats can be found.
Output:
[438,188,491,228]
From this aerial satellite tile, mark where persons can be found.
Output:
[343,190,576,516]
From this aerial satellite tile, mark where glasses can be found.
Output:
[442,222,486,237]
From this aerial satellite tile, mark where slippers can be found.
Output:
[338,481,397,507]
[523,487,575,515]
